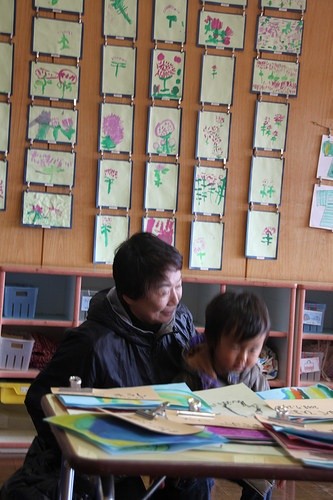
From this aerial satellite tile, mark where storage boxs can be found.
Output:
[0,382,31,404]
[300,352,324,382]
[3,286,39,319]
[0,337,35,371]
[303,303,326,333]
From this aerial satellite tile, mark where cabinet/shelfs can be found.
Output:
[79,289,98,321]
[0,263,333,489]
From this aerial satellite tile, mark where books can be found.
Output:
[46,383,333,469]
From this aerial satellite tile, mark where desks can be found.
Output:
[40,393,333,500]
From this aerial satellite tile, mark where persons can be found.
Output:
[173,291,275,500]
[0,233,199,500]
